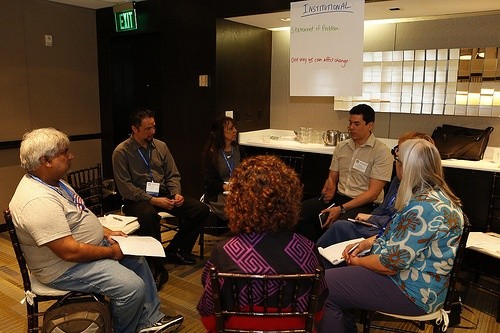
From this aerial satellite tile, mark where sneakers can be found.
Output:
[135,314,185,333]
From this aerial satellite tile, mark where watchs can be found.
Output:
[339,205,345,214]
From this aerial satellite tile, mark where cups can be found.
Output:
[336,131,351,142]
[323,130,338,145]
[298,126,326,144]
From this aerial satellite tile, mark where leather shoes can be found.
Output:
[153,265,169,292]
[165,241,197,265]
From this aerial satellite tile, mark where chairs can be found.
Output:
[363,214,469,333]
[210,268,323,333]
[473,173,500,322]
[4,208,113,333]
[68,153,306,260]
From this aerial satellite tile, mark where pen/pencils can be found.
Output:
[341,243,360,259]
[318,194,325,201]
[113,216,123,222]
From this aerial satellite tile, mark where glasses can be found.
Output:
[394,154,402,164]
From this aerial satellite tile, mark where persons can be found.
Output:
[314,132,464,333]
[197,154,329,333]
[201,117,245,222]
[8,127,184,333]
[112,110,209,291]
[292,104,393,243]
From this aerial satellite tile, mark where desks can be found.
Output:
[239,129,500,246]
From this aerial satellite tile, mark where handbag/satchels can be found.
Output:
[430,123,494,161]
